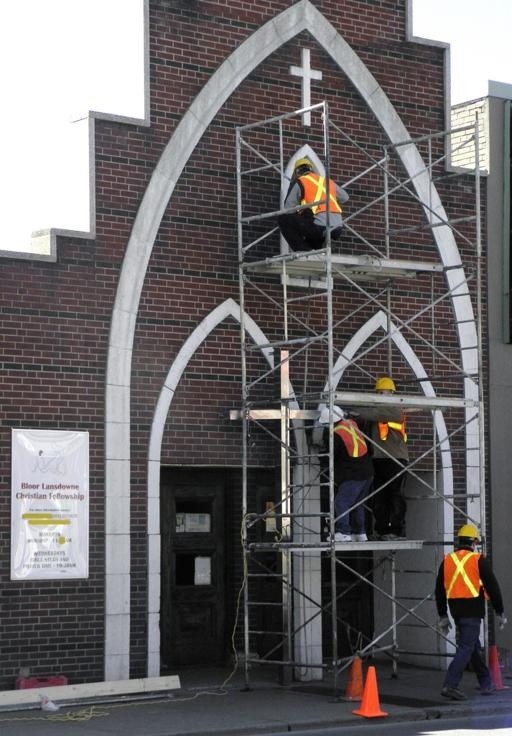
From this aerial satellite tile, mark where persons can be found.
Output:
[433,524,506,700]
[278,156,350,254]
[345,375,412,541]
[318,403,376,543]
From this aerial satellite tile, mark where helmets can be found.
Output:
[316,405,344,424]
[293,158,314,172]
[375,377,396,392]
[455,523,483,543]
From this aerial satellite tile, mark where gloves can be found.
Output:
[437,615,453,636]
[495,613,507,630]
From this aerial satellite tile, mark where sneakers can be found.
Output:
[350,532,367,542]
[438,685,467,702]
[478,686,497,696]
[381,532,407,541]
[367,532,381,542]
[326,531,352,543]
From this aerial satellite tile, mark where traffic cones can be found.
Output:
[486,644,509,691]
[343,652,363,703]
[352,664,388,720]
